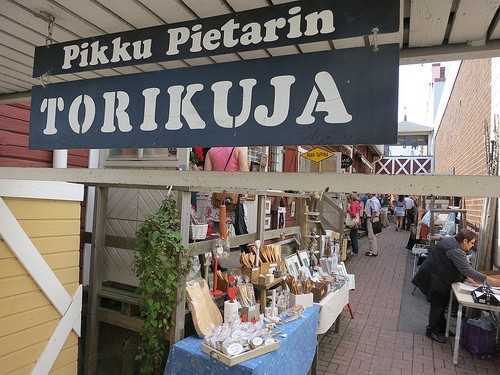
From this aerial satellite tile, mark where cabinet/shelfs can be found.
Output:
[303,212,324,254]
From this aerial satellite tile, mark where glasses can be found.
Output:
[469,241,475,246]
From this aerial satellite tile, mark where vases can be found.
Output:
[190,224,211,240]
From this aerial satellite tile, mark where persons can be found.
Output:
[410,229,500,343]
[380,194,419,232]
[347,194,362,256]
[201,147,250,272]
[364,194,380,257]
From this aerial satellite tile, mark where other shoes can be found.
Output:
[349,251,358,256]
[366,252,378,256]
[366,251,373,253]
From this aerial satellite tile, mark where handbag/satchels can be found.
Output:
[460,318,497,361]
[372,221,382,234]
[345,212,359,229]
[211,193,239,212]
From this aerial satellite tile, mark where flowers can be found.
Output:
[189,205,212,225]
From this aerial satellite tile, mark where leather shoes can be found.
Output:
[426,330,446,344]
[441,324,456,337]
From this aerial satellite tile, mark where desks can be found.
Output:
[270,272,355,342]
[444,282,499,365]
[163,304,321,375]
[412,245,428,277]
